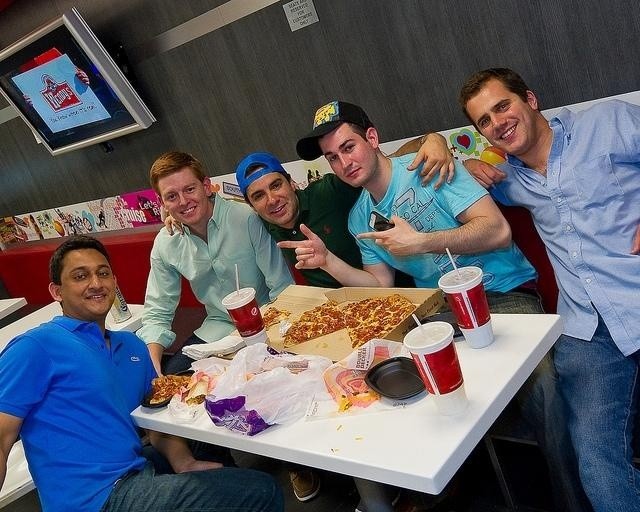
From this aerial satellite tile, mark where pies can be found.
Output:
[185,379,209,405]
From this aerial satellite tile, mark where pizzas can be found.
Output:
[283,294,416,353]
[150,374,190,404]
[263,307,290,328]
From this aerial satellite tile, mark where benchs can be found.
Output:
[0,200,558,312]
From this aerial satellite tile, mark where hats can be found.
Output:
[296,101,374,161]
[235,152,287,200]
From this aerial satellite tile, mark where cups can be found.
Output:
[437,265,496,350]
[222,287,268,348]
[402,321,467,411]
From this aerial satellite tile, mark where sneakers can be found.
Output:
[287,469,321,501]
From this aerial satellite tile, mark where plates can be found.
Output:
[142,372,195,409]
[364,356,427,400]
[419,309,461,338]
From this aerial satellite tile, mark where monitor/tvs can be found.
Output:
[0,6,158,157]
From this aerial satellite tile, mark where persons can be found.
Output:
[136,150,322,502]
[459,67,639,512]
[22,47,98,108]
[277,100,593,512]
[235,131,454,287]
[0,235,285,512]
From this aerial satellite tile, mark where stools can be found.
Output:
[0,439,36,509]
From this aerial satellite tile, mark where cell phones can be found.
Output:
[368,211,395,232]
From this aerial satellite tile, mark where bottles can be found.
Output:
[110,286,131,324]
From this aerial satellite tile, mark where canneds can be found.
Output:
[109,288,132,324]
